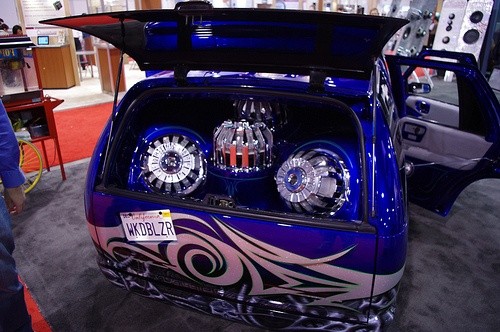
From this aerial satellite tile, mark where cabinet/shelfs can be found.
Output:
[35,43,76,89]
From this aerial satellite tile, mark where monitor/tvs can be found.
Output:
[37,35,58,46]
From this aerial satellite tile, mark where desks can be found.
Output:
[76,50,95,81]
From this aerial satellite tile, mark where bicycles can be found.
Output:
[11,120,43,195]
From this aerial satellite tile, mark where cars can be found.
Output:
[33,0,500,332]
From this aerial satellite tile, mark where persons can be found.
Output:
[370,8,378,16]
[357,6,361,13]
[0,96,33,332]
[13,25,22,34]
[0,18,9,34]
[492,32,500,70]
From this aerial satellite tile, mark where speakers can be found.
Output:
[430,0,494,63]
